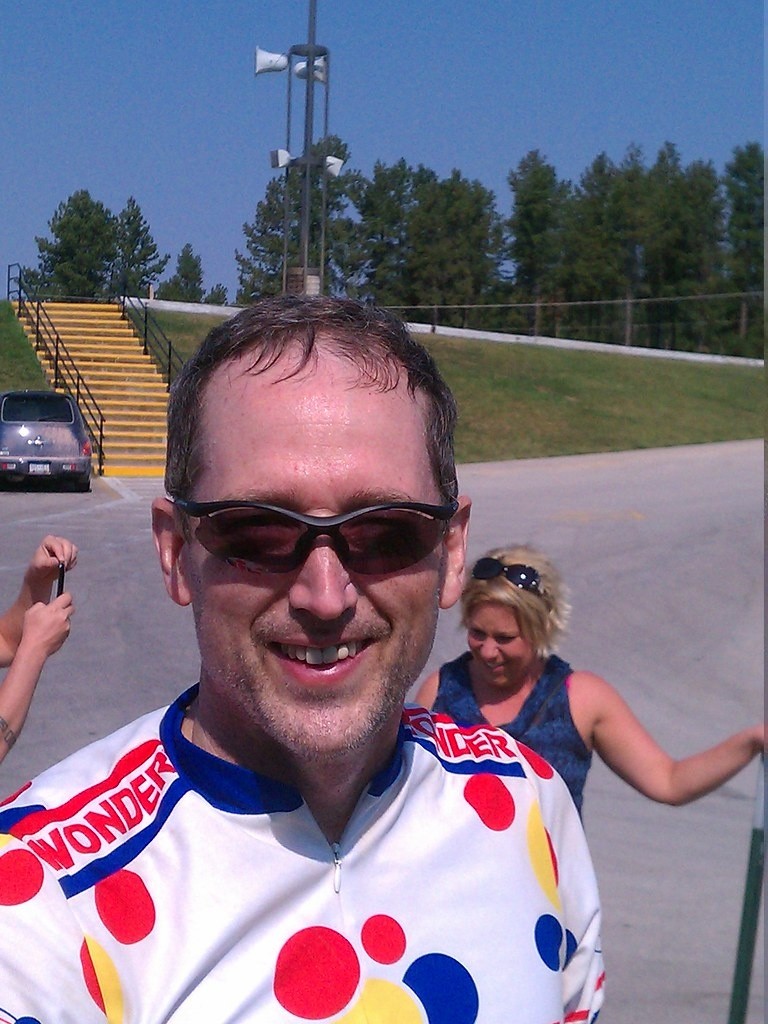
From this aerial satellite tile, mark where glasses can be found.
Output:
[473,556,551,600]
[167,496,459,575]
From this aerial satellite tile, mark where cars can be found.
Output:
[0,388,93,493]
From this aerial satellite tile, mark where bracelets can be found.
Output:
[0,716,17,747]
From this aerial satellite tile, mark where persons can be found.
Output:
[0,295,607,1024]
[415,545,765,824]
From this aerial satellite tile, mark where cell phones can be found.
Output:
[57,563,64,598]
